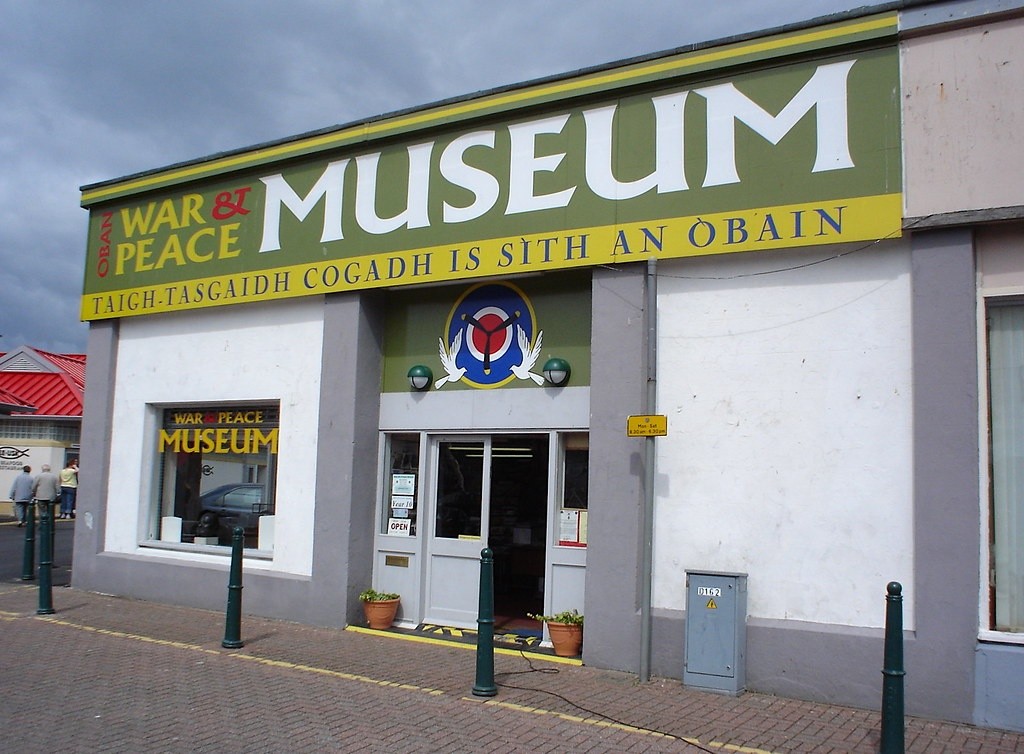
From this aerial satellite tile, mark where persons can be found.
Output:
[59,456,81,520]
[32,463,60,531]
[9,466,34,526]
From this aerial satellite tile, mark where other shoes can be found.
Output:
[17,521,22,526]
[61,514,72,519]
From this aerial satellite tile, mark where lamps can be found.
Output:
[407,365,433,392]
[543,358,571,387]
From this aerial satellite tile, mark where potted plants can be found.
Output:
[527,608,584,658]
[359,589,401,630]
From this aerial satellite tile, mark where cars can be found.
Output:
[200,483,265,532]
